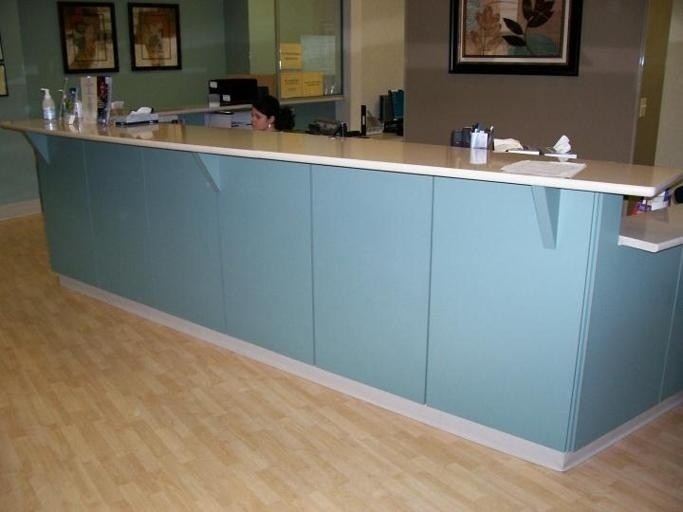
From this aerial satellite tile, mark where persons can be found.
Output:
[251,95,295,132]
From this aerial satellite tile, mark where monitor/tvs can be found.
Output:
[309,116,346,136]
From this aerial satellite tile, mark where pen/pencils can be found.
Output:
[469,122,487,133]
[488,125,496,144]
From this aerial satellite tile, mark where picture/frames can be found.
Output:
[447,0,583,77]
[56,1,182,76]
